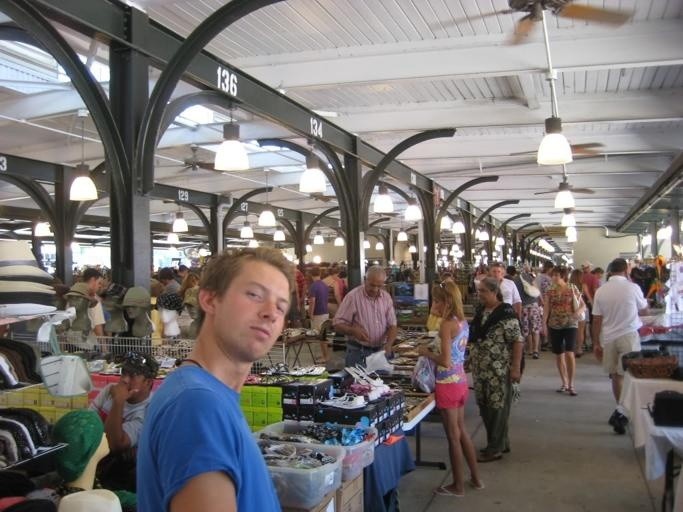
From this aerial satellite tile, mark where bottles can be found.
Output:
[323,417,369,445]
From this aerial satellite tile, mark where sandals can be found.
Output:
[568,387,578,395]
[559,385,569,392]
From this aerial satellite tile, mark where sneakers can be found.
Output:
[478,452,502,463]
[532,352,540,358]
[608,409,629,434]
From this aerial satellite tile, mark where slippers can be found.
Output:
[467,479,485,491]
[259,361,326,385]
[320,363,393,409]
[435,483,467,497]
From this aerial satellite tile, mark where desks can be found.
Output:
[275,335,346,367]
[616,371,683,511]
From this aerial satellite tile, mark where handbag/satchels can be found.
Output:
[411,355,437,393]
[573,295,585,322]
[522,282,540,297]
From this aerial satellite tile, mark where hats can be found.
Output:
[62,282,202,310]
[0,339,45,391]
[0,240,58,317]
[581,260,593,268]
[0,408,59,468]
[115,351,158,380]
[0,472,124,512]
[51,408,105,483]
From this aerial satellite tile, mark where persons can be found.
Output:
[50,283,202,354]
[36,408,136,511]
[330,264,397,380]
[135,247,300,512]
[478,261,601,395]
[418,277,484,498]
[85,350,158,489]
[468,275,524,462]
[592,258,651,405]
[628,254,670,307]
[63,254,191,337]
[293,259,348,363]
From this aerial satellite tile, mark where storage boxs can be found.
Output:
[2,374,163,428]
[236,375,404,512]
